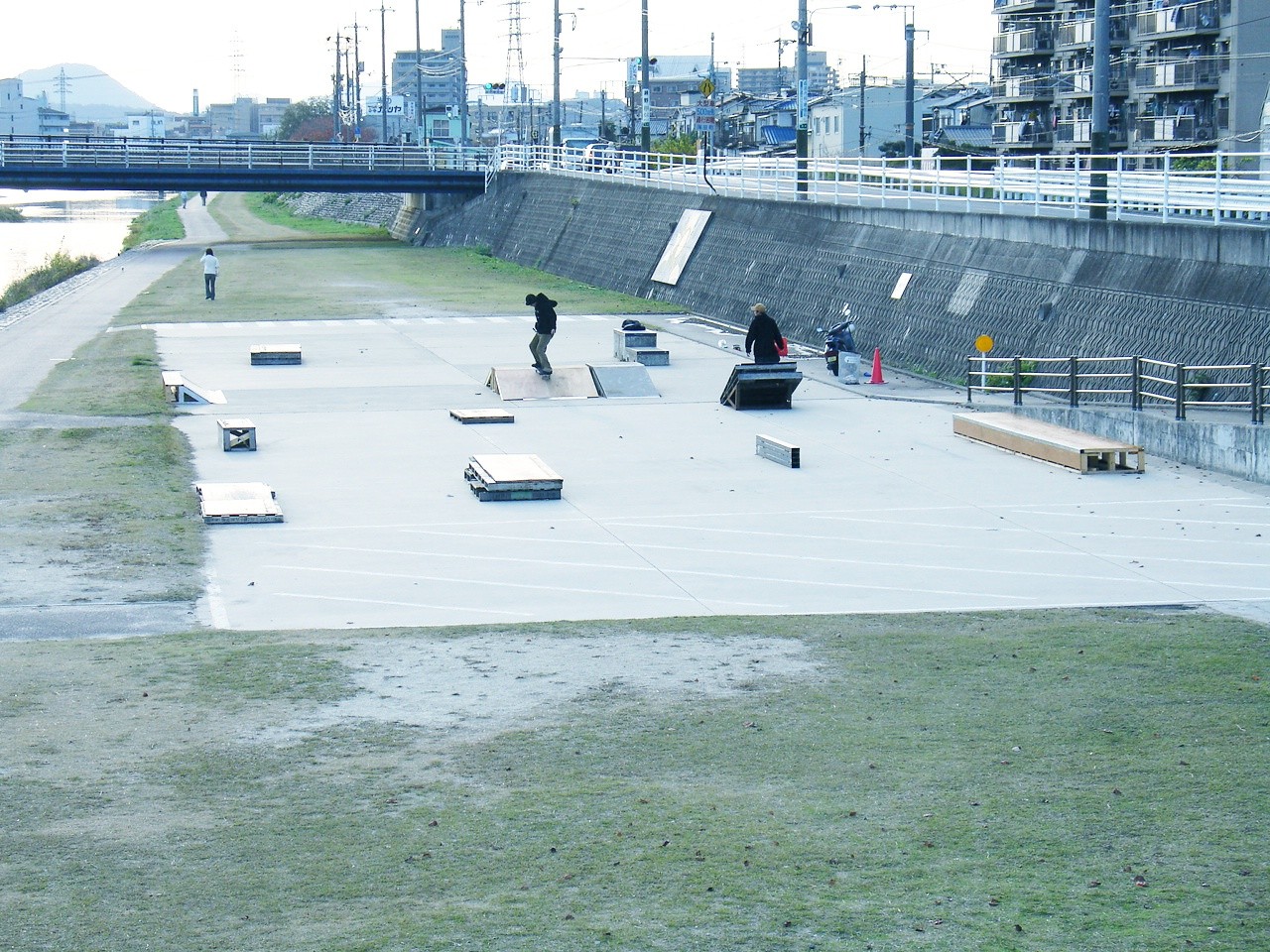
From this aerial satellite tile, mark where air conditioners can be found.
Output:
[1110,78,1123,92]
[1196,127,1213,140]
[1110,130,1122,143]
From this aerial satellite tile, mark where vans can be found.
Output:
[562,137,607,171]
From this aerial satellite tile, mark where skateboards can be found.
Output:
[535,366,551,380]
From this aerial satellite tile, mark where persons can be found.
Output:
[525,292,557,376]
[180,191,189,208]
[200,191,207,206]
[604,141,619,171]
[200,248,219,300]
[745,303,784,364]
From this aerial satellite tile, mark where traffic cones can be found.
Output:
[865,349,884,385]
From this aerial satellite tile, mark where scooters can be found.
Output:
[815,321,858,376]
[606,156,618,173]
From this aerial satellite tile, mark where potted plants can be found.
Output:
[1030,111,1038,121]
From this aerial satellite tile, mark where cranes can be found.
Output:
[24,67,109,114]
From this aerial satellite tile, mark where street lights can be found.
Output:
[789,5,863,200]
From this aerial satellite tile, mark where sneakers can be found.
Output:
[531,362,541,368]
[536,367,552,375]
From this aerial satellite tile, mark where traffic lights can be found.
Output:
[484,83,504,89]
[635,56,657,67]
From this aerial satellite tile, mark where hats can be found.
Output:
[525,294,536,305]
[751,303,766,313]
